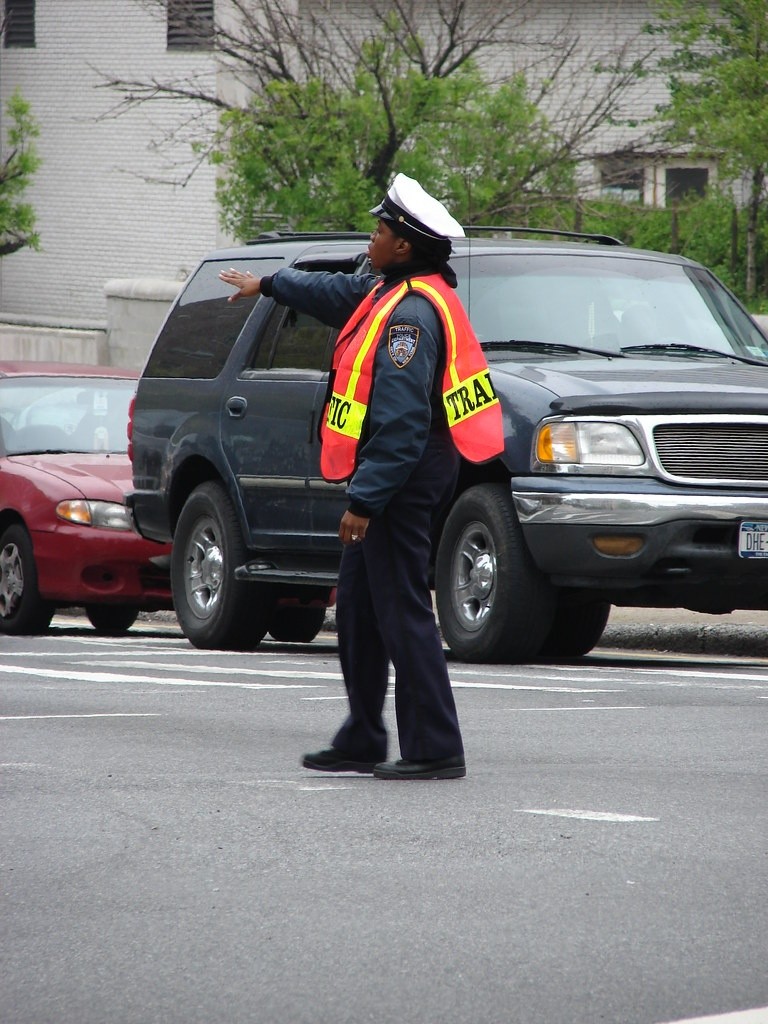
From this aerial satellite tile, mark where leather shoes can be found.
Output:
[302,741,388,774]
[374,756,465,780]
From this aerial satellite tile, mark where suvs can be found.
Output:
[129,225,768,661]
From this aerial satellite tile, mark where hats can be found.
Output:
[369,173,465,241]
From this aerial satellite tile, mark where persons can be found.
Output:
[219,172,505,780]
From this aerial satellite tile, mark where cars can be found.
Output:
[1,359,326,644]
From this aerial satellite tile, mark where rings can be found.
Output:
[352,535,358,540]
